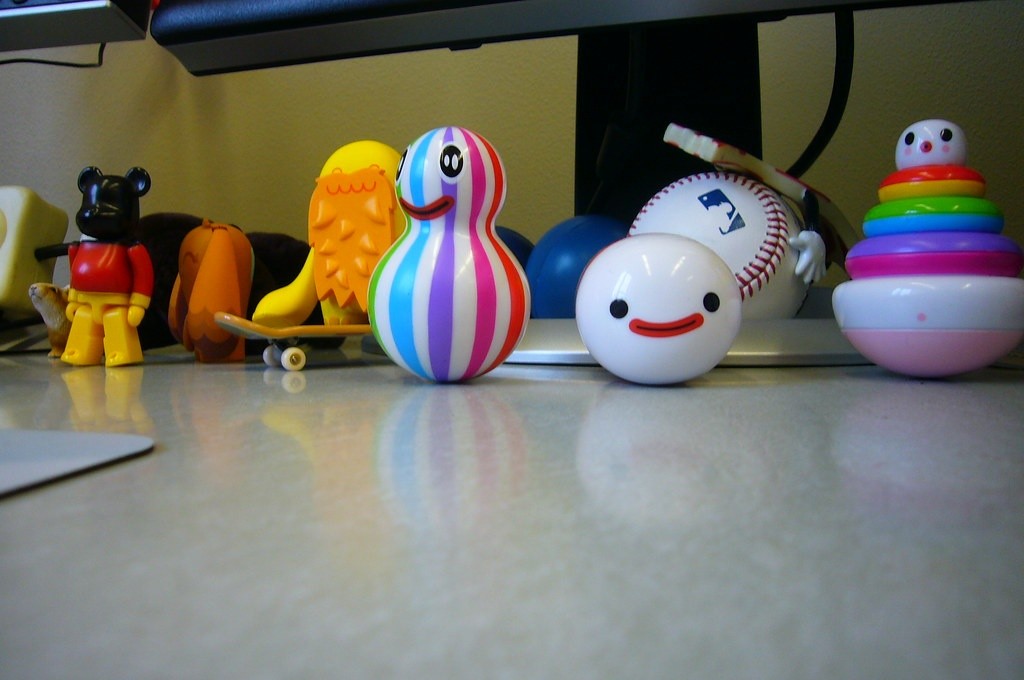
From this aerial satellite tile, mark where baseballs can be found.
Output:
[625,168,811,323]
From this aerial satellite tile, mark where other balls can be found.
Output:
[493,223,537,271]
[526,213,629,321]
